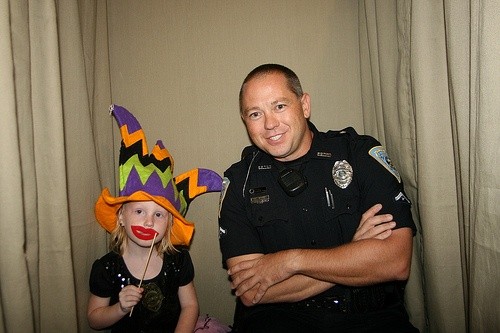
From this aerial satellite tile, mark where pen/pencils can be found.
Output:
[324,186,330,207]
[328,190,335,210]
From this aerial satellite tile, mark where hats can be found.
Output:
[94,104,226,247]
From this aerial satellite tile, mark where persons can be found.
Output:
[218,62,422,333]
[86,103,228,333]
